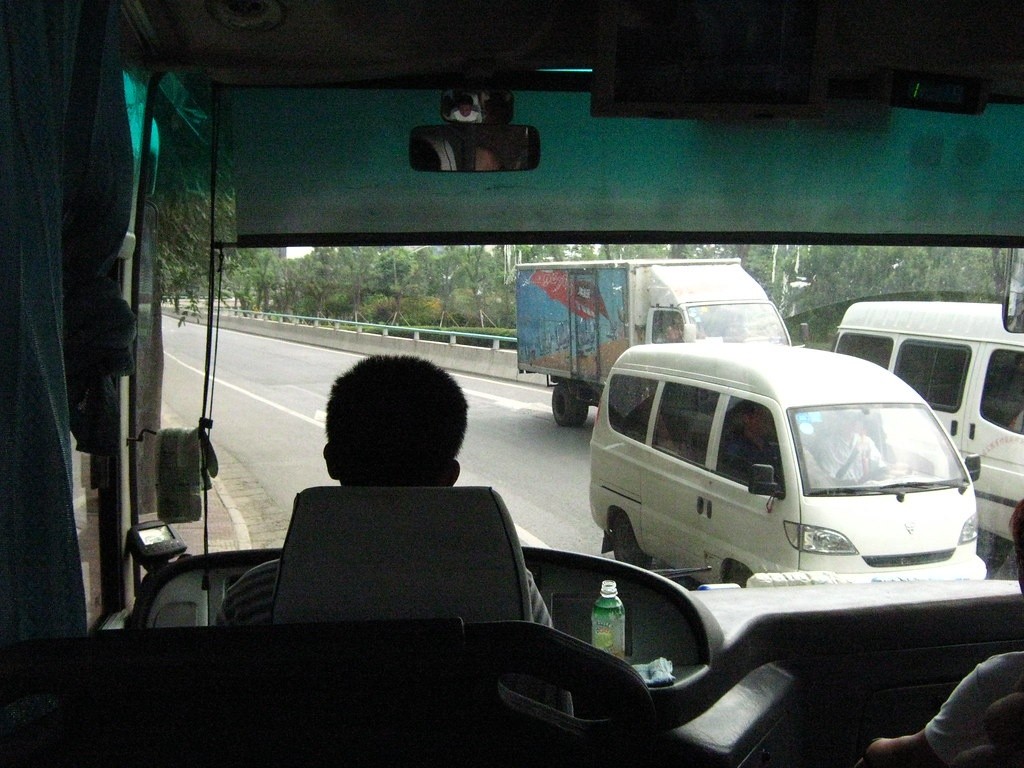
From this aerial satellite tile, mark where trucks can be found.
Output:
[512,256,812,428]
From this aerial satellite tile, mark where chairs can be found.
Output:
[951,692,1024,768]
[420,134,499,171]
[267,487,534,625]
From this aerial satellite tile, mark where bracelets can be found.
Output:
[862,738,878,768]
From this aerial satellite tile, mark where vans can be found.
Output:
[587,338,989,587]
[834,299,1024,546]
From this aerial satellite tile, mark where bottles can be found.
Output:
[591,580,625,662]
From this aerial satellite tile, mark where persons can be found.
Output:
[451,94,482,124]
[658,320,683,342]
[218,355,574,721]
[727,403,781,480]
[813,409,889,483]
[855,500,1024,768]
[410,126,529,173]
